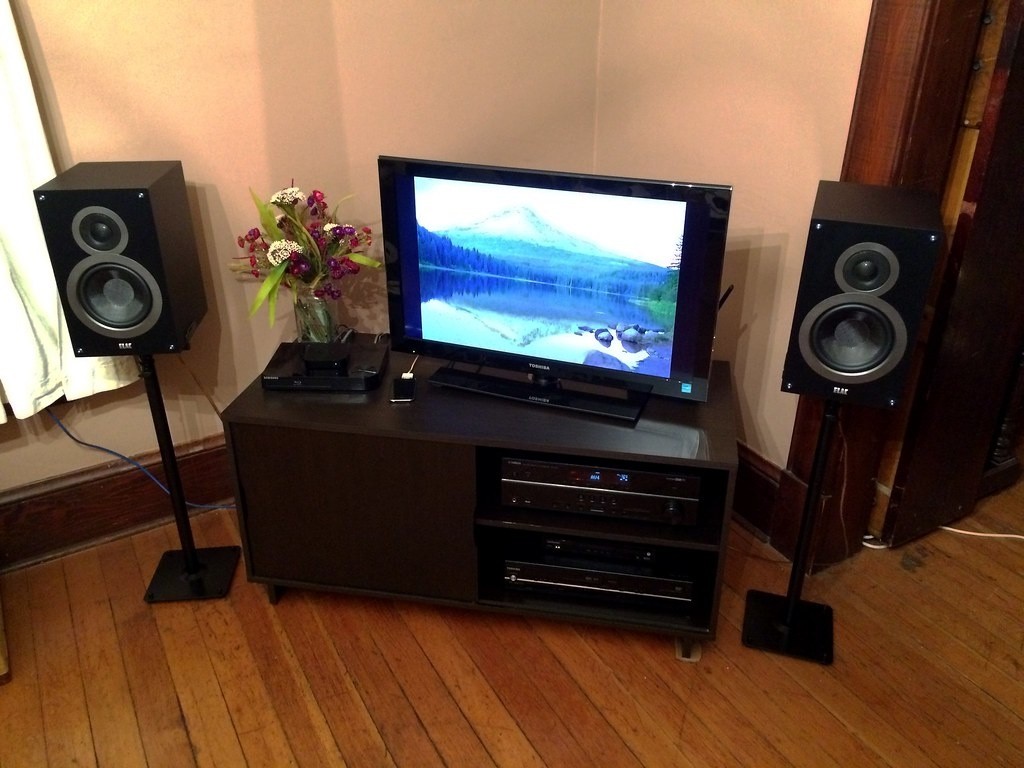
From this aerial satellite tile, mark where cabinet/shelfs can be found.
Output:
[220,332,741,658]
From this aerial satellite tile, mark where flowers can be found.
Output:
[227,177,383,342]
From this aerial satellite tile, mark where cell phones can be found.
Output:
[390,376,416,403]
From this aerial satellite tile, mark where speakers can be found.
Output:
[33,160,208,358]
[781,180,943,408]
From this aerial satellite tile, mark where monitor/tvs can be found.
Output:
[377,156,733,428]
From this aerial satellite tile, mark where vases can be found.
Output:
[292,283,342,343]
